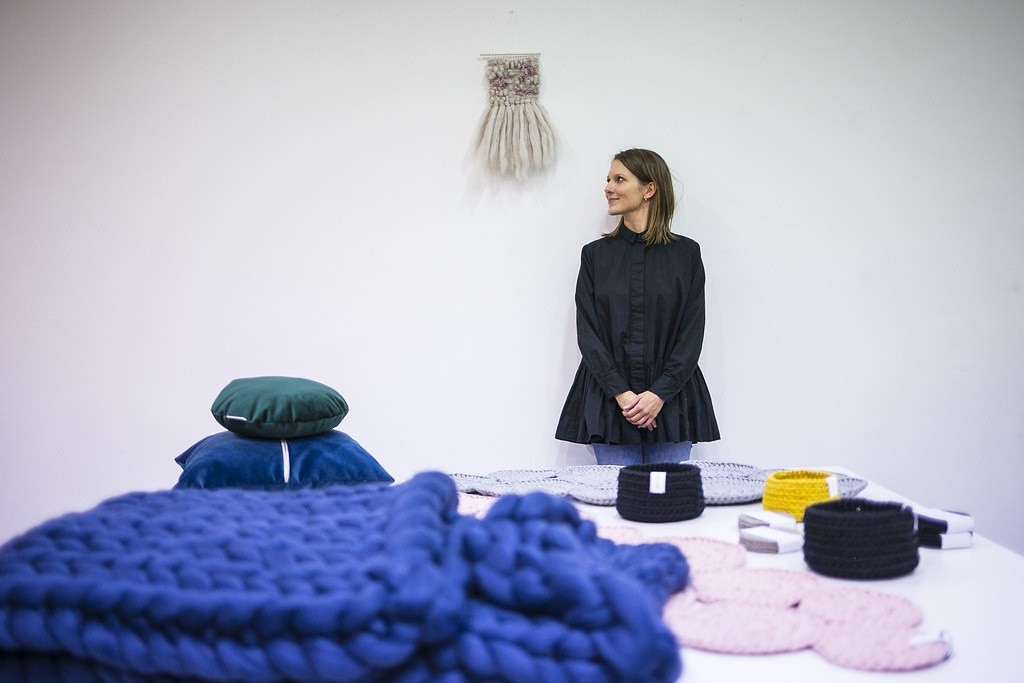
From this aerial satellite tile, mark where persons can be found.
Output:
[554,148,721,466]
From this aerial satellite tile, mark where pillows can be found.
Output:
[171,431,396,488]
[211,376,350,438]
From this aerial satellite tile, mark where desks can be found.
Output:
[446,467,1022,683]
